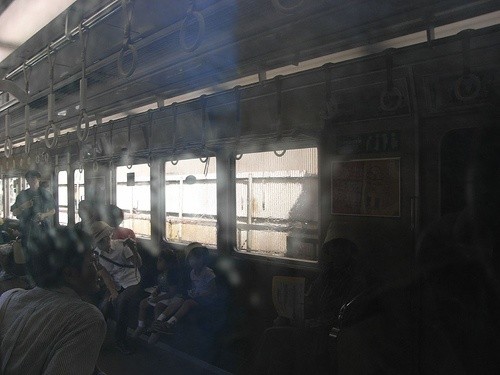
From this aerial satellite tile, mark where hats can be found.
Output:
[88,221,114,247]
[184,242,208,265]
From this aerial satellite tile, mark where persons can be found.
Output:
[0,171,217,355]
[0,228,109,375]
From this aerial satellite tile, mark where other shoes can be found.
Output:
[114,342,134,354]
[152,320,174,335]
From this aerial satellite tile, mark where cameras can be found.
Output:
[123,238,129,247]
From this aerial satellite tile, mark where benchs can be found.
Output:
[0,218,390,374]
